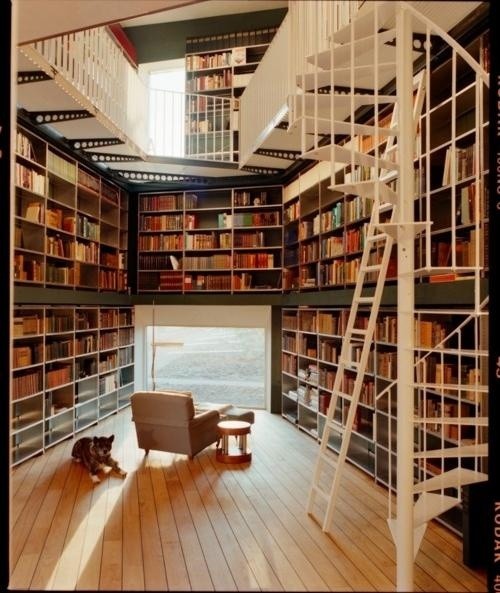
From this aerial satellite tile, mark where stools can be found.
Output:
[226,407,255,449]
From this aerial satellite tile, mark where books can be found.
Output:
[412,144,474,226]
[282,165,396,287]
[11,309,135,401]
[283,309,375,433]
[375,315,481,441]
[138,192,281,291]
[15,132,122,289]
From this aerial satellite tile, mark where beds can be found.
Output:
[216,420,252,464]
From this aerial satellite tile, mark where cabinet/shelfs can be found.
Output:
[15,116,131,290]
[283,10,489,293]
[279,306,490,537]
[138,185,284,293]
[12,303,135,469]
[183,41,269,163]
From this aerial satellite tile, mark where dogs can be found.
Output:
[71,435,128,484]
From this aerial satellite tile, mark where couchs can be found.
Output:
[130,390,228,461]
